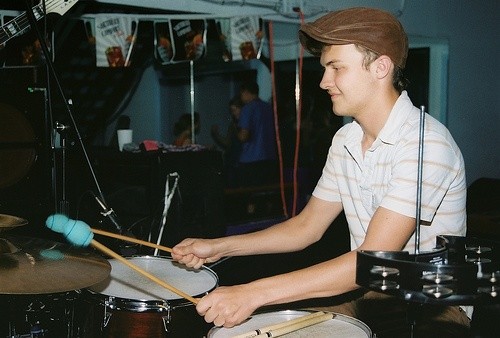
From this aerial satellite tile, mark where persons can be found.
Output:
[228,78,278,165]
[171,7,473,338]
[173,111,215,150]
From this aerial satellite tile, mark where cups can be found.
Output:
[117,129,133,151]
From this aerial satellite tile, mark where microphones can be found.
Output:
[96,197,137,245]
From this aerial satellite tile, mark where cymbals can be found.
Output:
[354,234,500,307]
[0,242,112,295]
[0,213,29,227]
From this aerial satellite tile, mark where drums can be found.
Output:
[207,307,373,338]
[66,255,219,338]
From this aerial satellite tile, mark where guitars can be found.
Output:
[0,0,80,46]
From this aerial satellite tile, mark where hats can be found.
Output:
[300,7,408,69]
